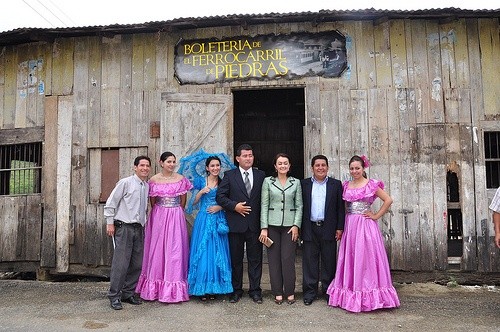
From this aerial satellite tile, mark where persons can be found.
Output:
[135,151,194,303]
[215,144,266,304]
[186,155,234,301]
[299,154,345,305]
[489,187,500,249]
[258,152,304,305]
[103,155,152,310]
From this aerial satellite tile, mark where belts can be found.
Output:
[122,222,142,227]
[311,221,324,226]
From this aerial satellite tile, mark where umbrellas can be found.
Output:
[177,147,238,210]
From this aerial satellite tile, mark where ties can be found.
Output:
[244,172,251,198]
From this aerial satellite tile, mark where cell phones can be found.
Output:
[263,237,274,247]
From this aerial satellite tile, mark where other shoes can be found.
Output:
[275,295,283,305]
[209,294,215,300]
[202,295,207,300]
[287,294,295,305]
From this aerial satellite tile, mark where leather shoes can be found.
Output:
[122,296,139,304]
[249,294,262,303]
[111,299,122,309]
[229,293,242,303]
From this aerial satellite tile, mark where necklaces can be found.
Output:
[326,155,401,313]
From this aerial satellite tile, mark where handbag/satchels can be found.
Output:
[217,210,229,235]
